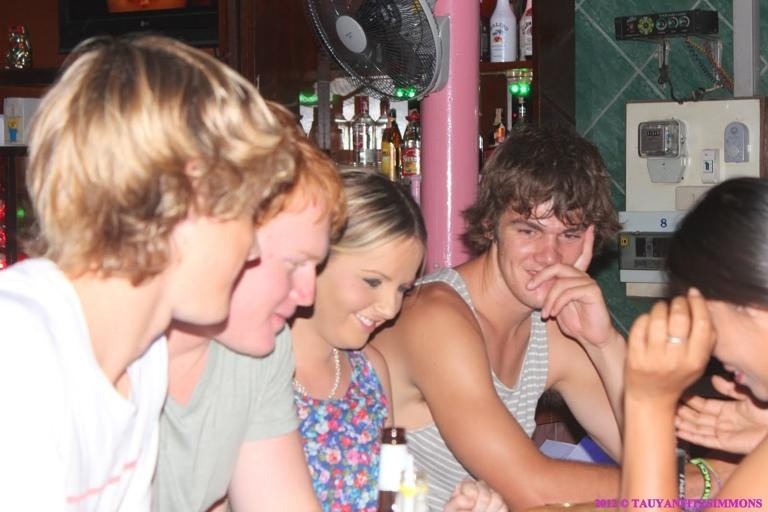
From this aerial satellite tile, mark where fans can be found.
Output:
[305,0,450,102]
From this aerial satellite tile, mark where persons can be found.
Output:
[616,178,767,512]
[294,164,513,511]
[157,94,351,512]
[378,122,733,512]
[0,32,296,512]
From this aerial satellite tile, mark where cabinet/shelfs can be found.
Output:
[480,0,574,172]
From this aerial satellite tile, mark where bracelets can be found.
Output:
[675,452,724,512]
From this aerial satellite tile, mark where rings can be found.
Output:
[666,334,689,345]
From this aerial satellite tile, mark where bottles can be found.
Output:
[375,426,429,512]
[5,24,33,70]
[489,0,518,63]
[489,97,529,146]
[518,0,533,62]
[329,94,421,188]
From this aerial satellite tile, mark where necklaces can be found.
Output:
[293,345,341,401]
